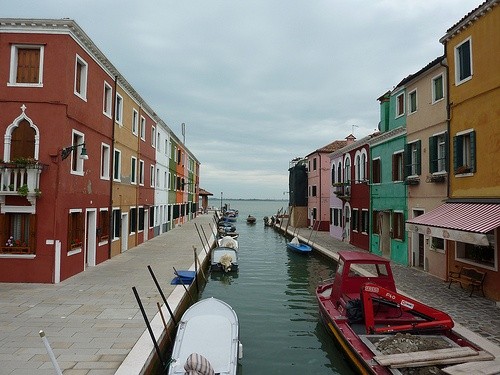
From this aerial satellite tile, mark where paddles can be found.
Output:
[209,222,216,241]
[172,266,194,301]
[211,211,220,229]
[306,219,322,248]
[194,222,212,260]
[38,330,62,375]
[191,244,206,296]
[156,301,173,347]
[292,215,306,237]
[131,285,166,368]
[148,264,179,323]
[275,207,294,236]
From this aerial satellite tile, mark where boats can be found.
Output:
[287,236,312,254]
[314,250,500,375]
[168,297,244,375]
[174,270,197,280]
[210,209,239,272]
[247,214,257,224]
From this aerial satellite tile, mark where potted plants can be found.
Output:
[0,155,36,167]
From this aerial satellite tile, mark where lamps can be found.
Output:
[178,183,198,189]
[62,141,88,162]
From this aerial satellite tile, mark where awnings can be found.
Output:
[404,203,500,246]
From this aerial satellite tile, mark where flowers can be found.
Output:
[71,239,80,245]
[5,236,27,247]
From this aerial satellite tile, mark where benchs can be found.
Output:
[447,266,487,297]
